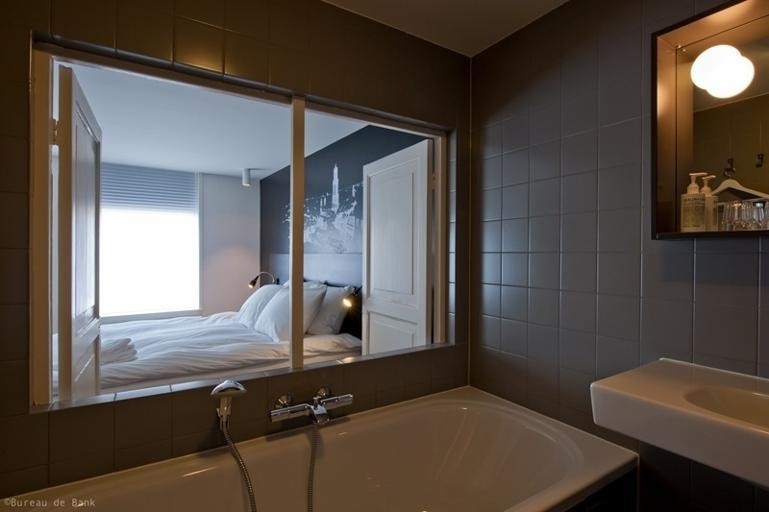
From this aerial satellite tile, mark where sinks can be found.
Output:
[589,357,769,491]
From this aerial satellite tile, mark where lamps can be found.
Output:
[343,286,362,307]
[242,168,251,186]
[248,272,279,288]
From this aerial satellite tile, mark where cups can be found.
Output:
[720,200,769,231]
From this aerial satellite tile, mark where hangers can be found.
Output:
[711,167,769,207]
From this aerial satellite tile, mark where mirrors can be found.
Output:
[651,0,769,240]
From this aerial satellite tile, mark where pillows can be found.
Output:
[233,279,355,342]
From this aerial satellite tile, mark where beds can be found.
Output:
[100,279,361,389]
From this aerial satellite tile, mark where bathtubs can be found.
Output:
[1,383,641,511]
